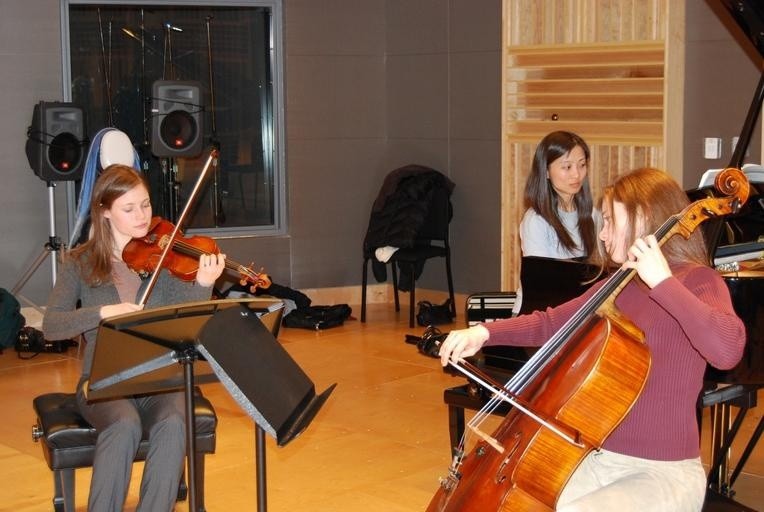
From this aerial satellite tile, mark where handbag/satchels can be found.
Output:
[405,324,449,359]
[13,327,79,359]
[416,297,454,327]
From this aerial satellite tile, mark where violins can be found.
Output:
[122,215,273,293]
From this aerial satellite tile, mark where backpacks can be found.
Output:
[282,303,353,331]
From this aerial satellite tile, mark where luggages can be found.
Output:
[465,292,517,329]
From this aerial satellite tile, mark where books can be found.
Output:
[696,162,764,184]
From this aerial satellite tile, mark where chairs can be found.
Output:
[360,165,456,328]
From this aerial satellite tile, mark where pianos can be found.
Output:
[483,180,764,494]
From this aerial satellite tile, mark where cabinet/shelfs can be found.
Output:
[502,0,682,291]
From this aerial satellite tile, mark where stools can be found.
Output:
[32,386,216,511]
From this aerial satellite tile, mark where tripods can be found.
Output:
[9,182,66,353]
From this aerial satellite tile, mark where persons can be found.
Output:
[510,130,603,318]
[40,164,226,511]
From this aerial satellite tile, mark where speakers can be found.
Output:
[139,79,204,158]
[25,101,85,182]
